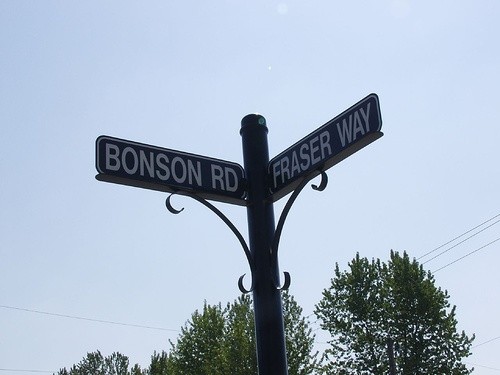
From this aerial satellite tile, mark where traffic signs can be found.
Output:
[96,135,248,203]
[266,93,382,196]
